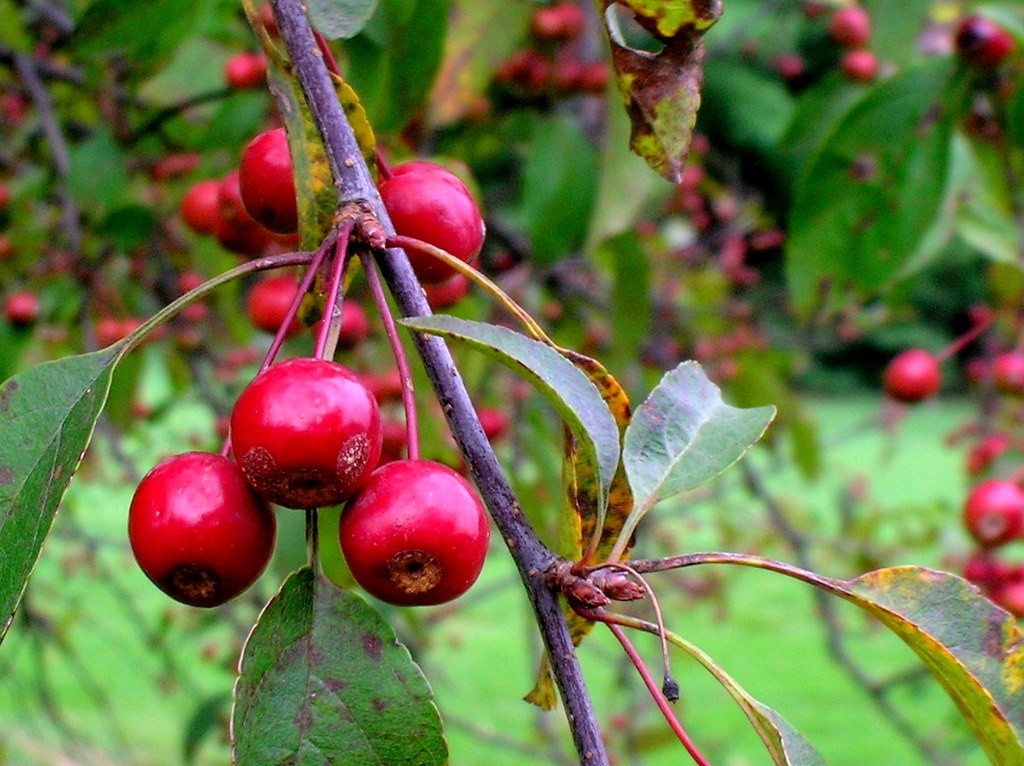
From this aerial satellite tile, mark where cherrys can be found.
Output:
[0,0,1024,609]
